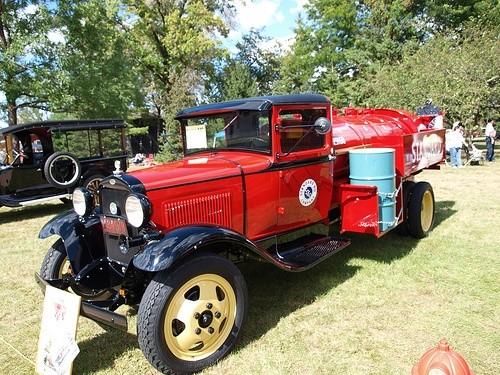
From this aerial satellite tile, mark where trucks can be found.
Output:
[39,93,446,375]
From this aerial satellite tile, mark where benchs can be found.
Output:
[464,141,485,167]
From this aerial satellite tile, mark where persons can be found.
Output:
[133,153,145,166]
[484,118,497,162]
[448,121,465,168]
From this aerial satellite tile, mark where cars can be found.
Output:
[0,121,129,213]
